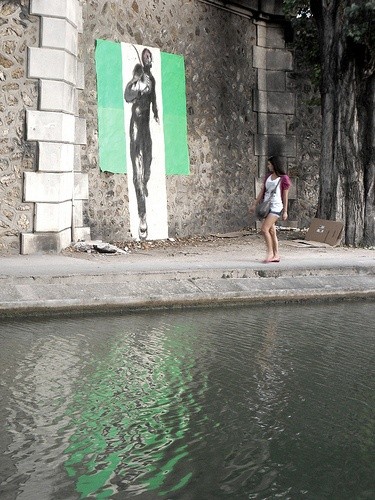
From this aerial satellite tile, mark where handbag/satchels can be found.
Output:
[257,201,271,219]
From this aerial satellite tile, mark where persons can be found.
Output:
[248,155,290,264]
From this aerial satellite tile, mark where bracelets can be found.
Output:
[252,205,256,208]
